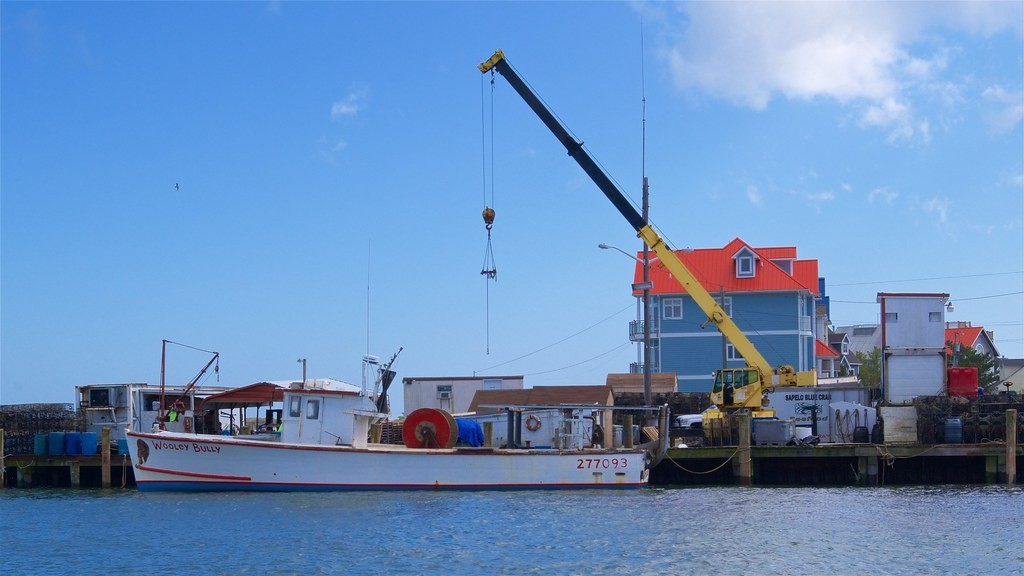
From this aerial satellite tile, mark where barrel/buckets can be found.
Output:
[32,432,98,456]
[944,418,963,444]
[117,438,129,455]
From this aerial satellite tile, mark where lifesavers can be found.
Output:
[524,415,540,432]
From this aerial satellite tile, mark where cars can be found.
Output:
[672,404,718,434]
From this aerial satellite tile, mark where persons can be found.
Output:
[169,398,185,422]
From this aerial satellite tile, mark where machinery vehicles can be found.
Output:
[476,49,817,448]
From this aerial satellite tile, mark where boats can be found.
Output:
[124,426,651,491]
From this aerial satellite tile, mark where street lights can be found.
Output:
[594,243,696,426]
